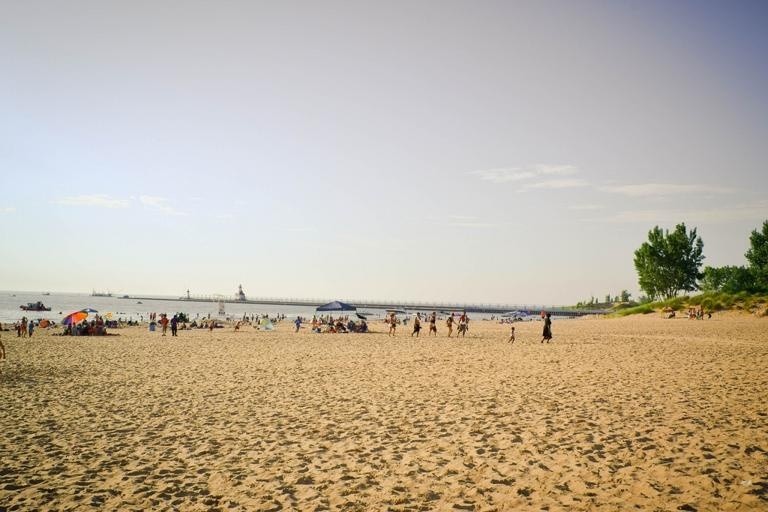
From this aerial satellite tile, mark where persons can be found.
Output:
[15,307,117,338]
[491,314,521,324]
[242,313,286,331]
[149,311,215,336]
[234,322,242,332]
[541,310,545,319]
[295,316,306,333]
[383,311,469,337]
[0,338,5,372]
[508,327,515,344]
[311,315,367,333]
[541,313,551,343]
[689,308,703,319]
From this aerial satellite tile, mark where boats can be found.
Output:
[40,291,50,296]
[18,301,51,312]
[90,292,112,297]
[354,306,558,323]
